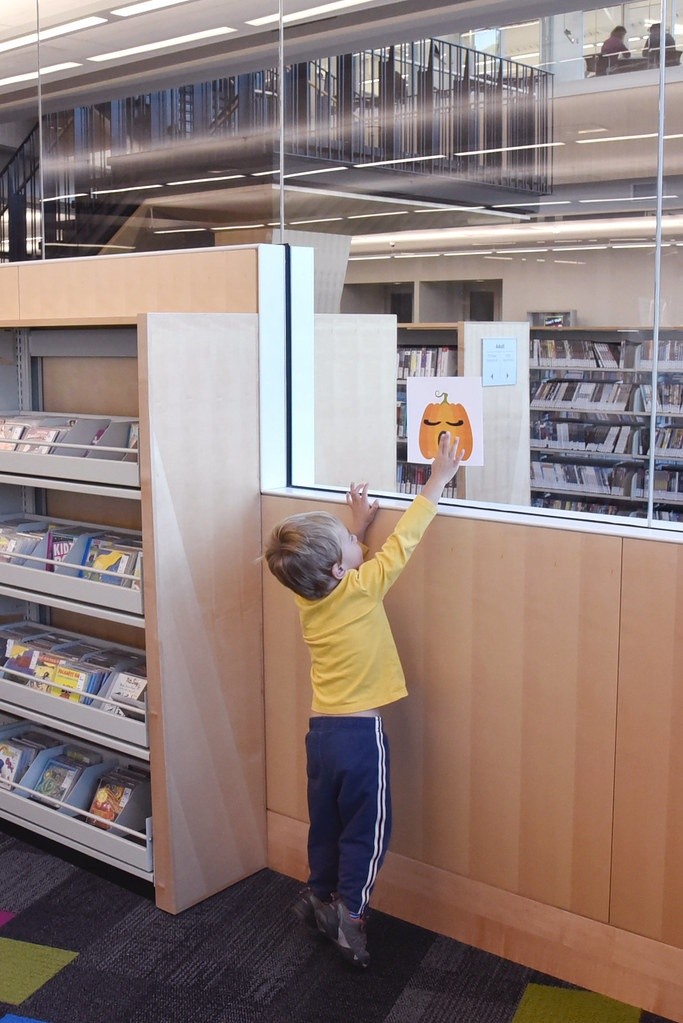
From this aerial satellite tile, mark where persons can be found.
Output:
[599,21,676,75]
[264,431,466,969]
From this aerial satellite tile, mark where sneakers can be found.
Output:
[294,884,335,942]
[314,894,372,967]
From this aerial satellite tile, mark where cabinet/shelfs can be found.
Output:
[528,326,683,507]
[396,320,531,507]
[0,311,267,916]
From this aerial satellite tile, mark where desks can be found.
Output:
[617,58,648,73]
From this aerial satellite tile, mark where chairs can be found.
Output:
[583,53,608,78]
[652,49,683,68]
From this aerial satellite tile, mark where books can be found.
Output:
[397,340,683,523]
[0,425,147,830]
